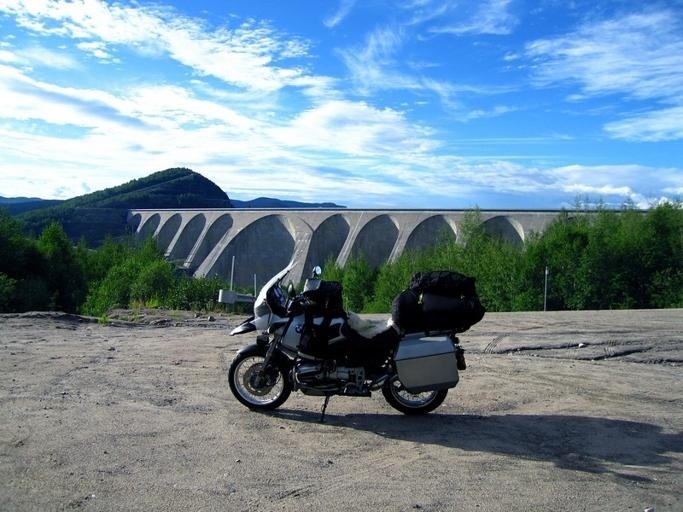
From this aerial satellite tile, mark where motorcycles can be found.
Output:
[223,262,466,421]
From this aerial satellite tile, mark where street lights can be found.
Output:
[542,266,549,312]
